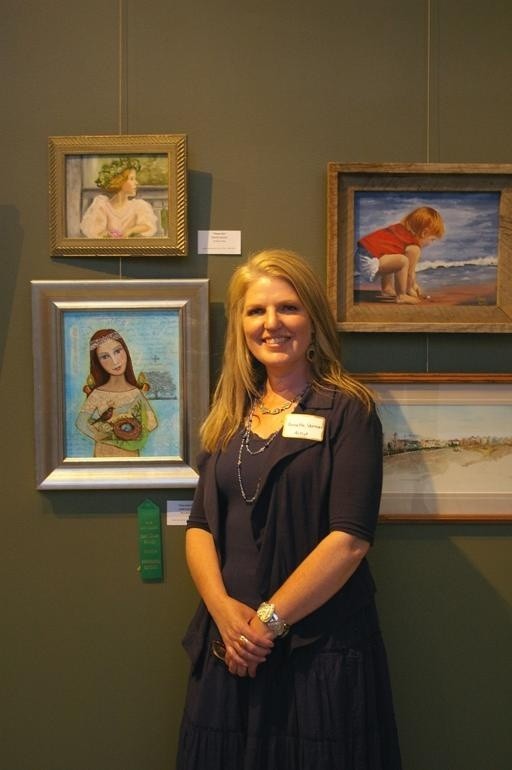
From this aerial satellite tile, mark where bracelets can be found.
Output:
[256,601,290,638]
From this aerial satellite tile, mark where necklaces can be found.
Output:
[237,383,310,504]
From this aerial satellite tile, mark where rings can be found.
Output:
[239,635,249,646]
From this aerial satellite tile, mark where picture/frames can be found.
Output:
[344,371,511,526]
[29,276,212,492]
[46,134,191,258]
[326,159,511,336]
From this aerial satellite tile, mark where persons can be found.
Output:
[354,205,444,304]
[79,156,159,239]
[74,329,159,458]
[169,248,403,770]
